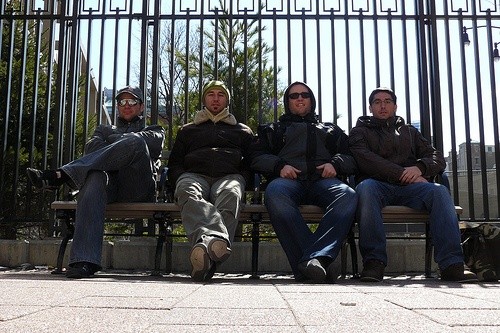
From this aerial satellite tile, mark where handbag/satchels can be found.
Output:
[460,223,500,281]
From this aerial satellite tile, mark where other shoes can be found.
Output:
[360,264,384,282]
[296,272,313,283]
[441,263,477,282]
[191,243,214,282]
[204,235,233,264]
[298,258,326,282]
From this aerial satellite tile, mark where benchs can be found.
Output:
[51,149,463,278]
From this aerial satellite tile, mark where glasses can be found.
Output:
[288,92,311,99]
[117,98,141,107]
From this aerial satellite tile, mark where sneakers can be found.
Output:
[65,261,92,279]
[26,167,62,192]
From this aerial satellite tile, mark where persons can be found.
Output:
[249,82,357,284]
[26,85,166,278]
[346,87,479,282]
[167,80,255,283]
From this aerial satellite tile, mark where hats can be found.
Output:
[203,80,231,106]
[116,86,144,103]
